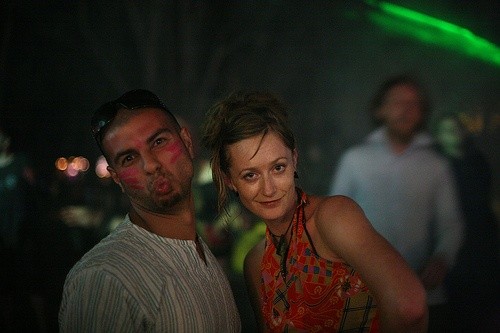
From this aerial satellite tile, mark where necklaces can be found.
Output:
[269,209,295,285]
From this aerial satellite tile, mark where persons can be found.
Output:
[58,89,242,333]
[203,85,429,333]
[328,75,466,285]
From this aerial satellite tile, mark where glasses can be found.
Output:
[91,89,182,163]
